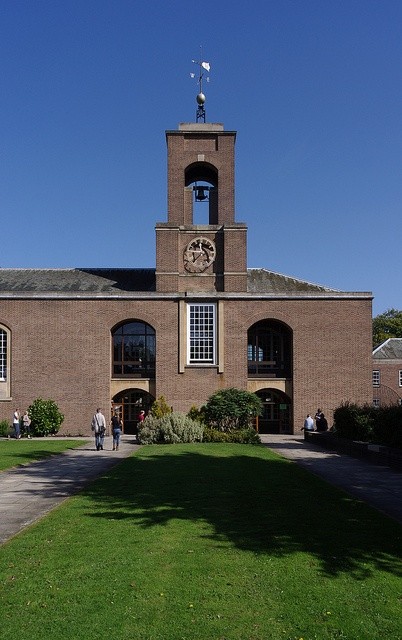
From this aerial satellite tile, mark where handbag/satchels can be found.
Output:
[94,414,106,438]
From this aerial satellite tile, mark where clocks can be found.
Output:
[185,236,217,268]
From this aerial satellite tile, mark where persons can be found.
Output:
[109,411,123,450]
[91,408,106,451]
[13,408,20,439]
[22,411,32,439]
[315,413,328,431]
[301,414,314,431]
[314,408,324,429]
[138,410,146,423]
